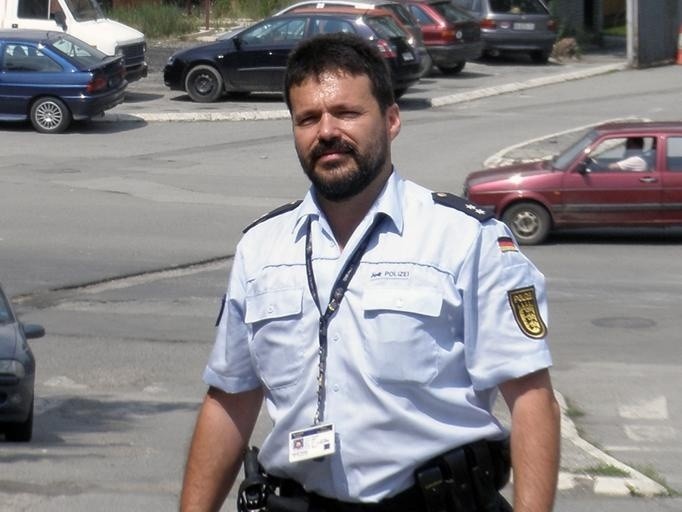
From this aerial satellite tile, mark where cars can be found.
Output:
[374,0,484,74]
[451,0,556,65]
[463,121,682,246]
[271,0,432,81]
[0,29,128,133]
[0,289,46,443]
[165,8,424,103]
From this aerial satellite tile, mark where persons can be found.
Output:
[178,31,561,512]
[595,138,648,171]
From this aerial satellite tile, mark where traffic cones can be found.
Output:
[676,27,682,65]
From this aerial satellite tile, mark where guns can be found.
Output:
[237,444,269,512]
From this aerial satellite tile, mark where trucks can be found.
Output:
[0,0,148,84]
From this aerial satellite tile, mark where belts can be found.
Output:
[242,443,513,512]
